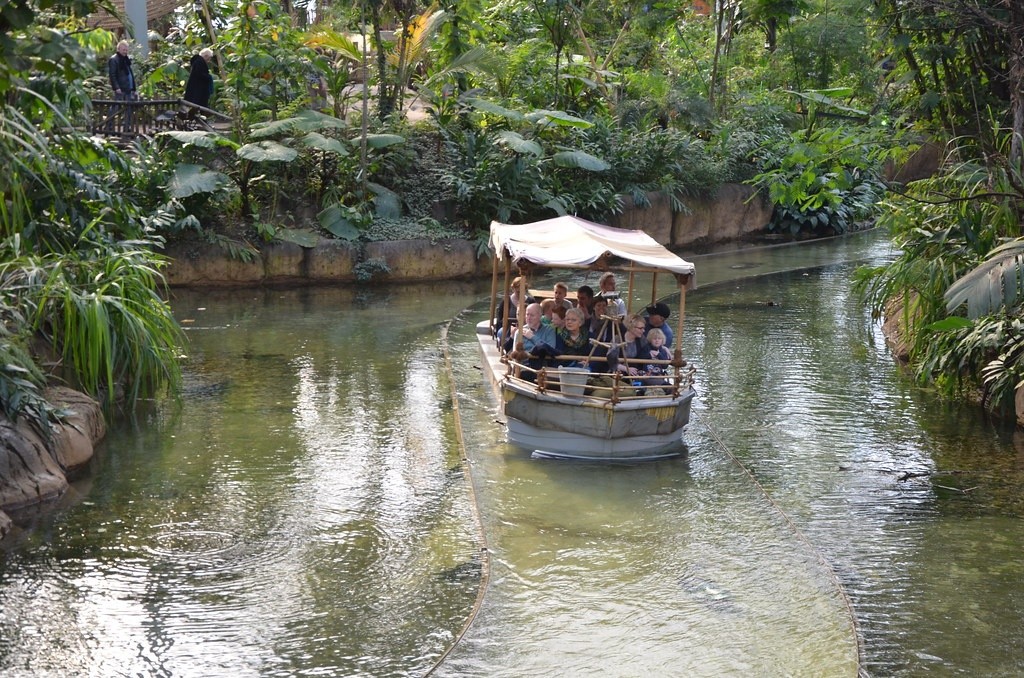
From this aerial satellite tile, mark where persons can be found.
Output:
[495,272,674,396]
[106,39,135,136]
[183,47,215,119]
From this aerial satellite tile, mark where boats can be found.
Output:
[475,214,699,465]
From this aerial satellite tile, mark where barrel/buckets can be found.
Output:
[557,364,591,395]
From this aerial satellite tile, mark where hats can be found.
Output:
[647,303,670,318]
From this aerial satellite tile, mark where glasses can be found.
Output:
[564,318,581,322]
[634,326,645,330]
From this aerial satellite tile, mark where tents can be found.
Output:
[487,214,697,373]
